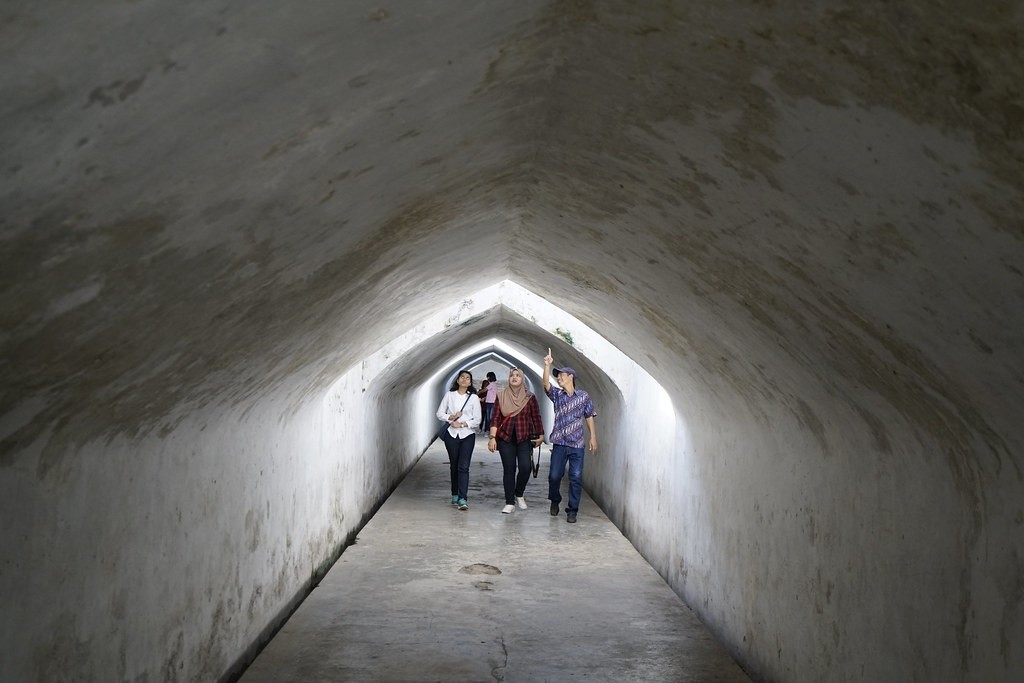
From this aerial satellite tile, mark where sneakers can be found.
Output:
[452,494,459,506]
[477,429,489,437]
[457,498,468,510]
[515,495,527,509]
[502,505,515,513]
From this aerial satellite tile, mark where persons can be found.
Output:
[479,371,499,439]
[543,347,598,524]
[435,370,482,510]
[488,367,545,515]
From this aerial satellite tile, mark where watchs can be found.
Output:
[488,435,495,439]
[460,422,464,428]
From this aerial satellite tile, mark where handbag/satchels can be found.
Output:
[495,432,500,450]
[437,422,450,441]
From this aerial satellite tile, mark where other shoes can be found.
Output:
[567,512,577,523]
[550,501,559,515]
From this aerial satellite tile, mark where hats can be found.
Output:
[552,367,577,380]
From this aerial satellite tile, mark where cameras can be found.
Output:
[528,433,539,447]
